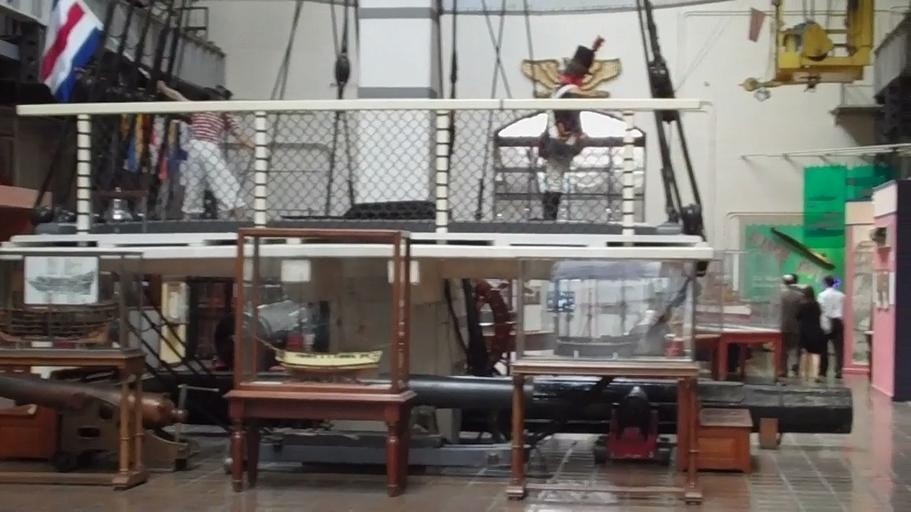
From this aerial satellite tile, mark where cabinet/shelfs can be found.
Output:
[870,179,911,403]
[505,251,714,507]
[228,223,420,498]
[838,197,874,379]
[3,248,152,494]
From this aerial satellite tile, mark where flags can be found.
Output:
[36,1,106,101]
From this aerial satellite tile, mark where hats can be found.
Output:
[566,36,605,76]
[205,85,232,99]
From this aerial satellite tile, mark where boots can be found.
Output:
[531,193,560,220]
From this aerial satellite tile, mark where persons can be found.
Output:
[535,134,589,222]
[151,79,258,220]
[780,272,847,382]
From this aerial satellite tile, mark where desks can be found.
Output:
[696,319,787,382]
